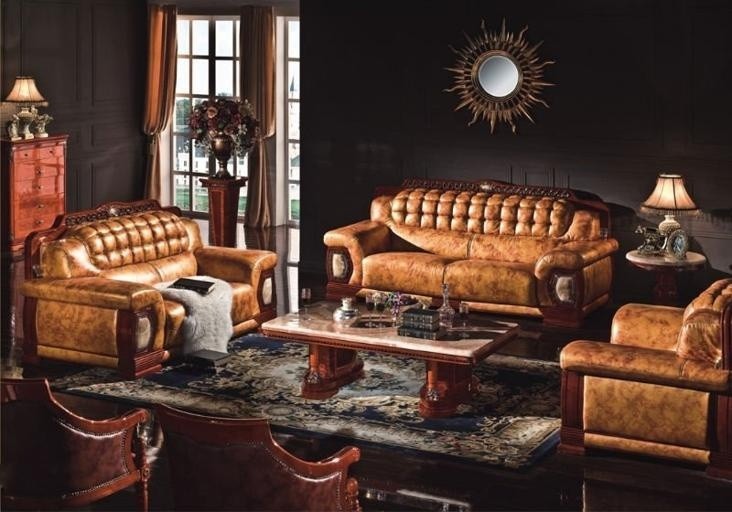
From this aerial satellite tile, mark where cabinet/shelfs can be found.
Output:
[2,130,72,262]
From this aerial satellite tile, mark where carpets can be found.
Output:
[46,331,561,474]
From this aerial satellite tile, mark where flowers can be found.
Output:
[188,94,259,160]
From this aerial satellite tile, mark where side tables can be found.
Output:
[626,240,707,303]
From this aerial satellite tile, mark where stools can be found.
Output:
[197,176,249,248]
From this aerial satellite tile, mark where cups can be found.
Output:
[301,287,312,322]
[365,295,375,326]
[376,296,385,324]
[459,303,469,329]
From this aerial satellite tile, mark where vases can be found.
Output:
[205,130,237,182]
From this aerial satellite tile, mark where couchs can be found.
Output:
[321,172,621,330]
[17,195,280,380]
[556,277,732,480]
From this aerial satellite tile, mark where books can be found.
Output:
[401,308,441,323]
[401,319,442,331]
[396,326,449,340]
[170,277,217,295]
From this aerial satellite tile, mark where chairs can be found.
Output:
[0,365,156,512]
[156,395,362,512]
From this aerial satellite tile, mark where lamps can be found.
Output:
[640,172,701,264]
[1,74,53,139]
